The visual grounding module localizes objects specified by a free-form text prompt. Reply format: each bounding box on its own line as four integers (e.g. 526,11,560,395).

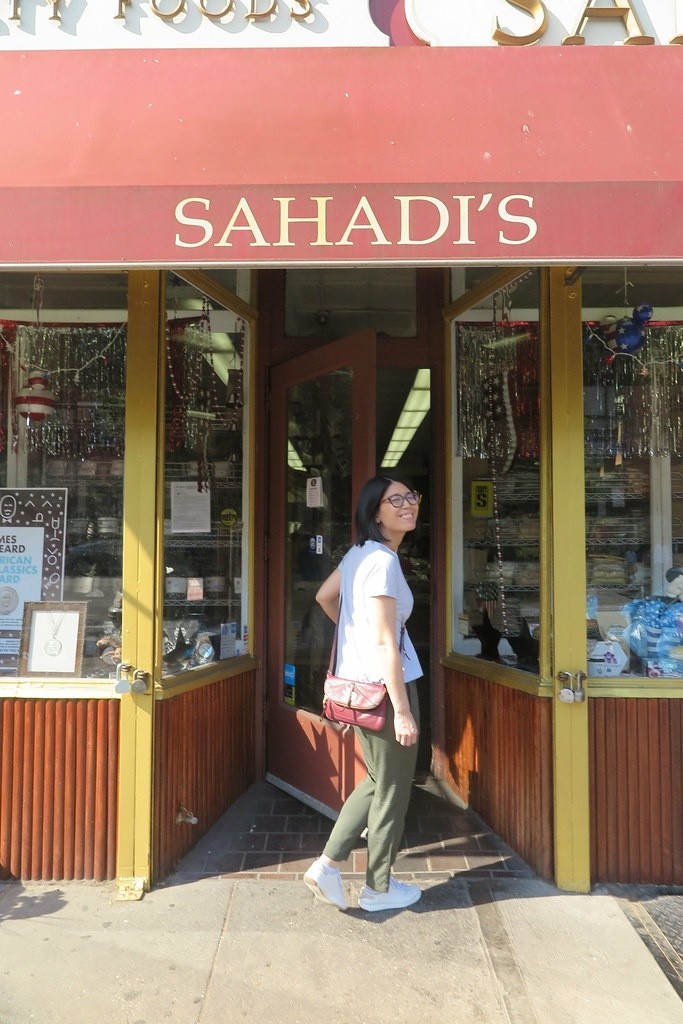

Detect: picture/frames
17,601,89,677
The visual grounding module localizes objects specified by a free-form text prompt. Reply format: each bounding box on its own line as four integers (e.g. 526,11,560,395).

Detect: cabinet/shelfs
36,458,683,636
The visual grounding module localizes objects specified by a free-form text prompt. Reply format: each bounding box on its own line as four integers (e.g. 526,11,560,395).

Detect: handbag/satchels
319,675,389,732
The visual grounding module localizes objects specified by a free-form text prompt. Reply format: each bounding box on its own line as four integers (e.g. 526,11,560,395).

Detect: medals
43,637,62,657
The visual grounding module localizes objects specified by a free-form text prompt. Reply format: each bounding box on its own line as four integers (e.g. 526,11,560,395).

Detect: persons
303,473,424,912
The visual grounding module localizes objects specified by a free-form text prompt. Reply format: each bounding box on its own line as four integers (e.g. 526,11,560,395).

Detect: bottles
43,456,240,602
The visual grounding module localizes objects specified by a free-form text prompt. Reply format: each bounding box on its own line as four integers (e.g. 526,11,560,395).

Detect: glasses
379,491,421,508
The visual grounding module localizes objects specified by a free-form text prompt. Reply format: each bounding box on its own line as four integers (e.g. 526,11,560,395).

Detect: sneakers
302,861,347,911
358,876,422,912
